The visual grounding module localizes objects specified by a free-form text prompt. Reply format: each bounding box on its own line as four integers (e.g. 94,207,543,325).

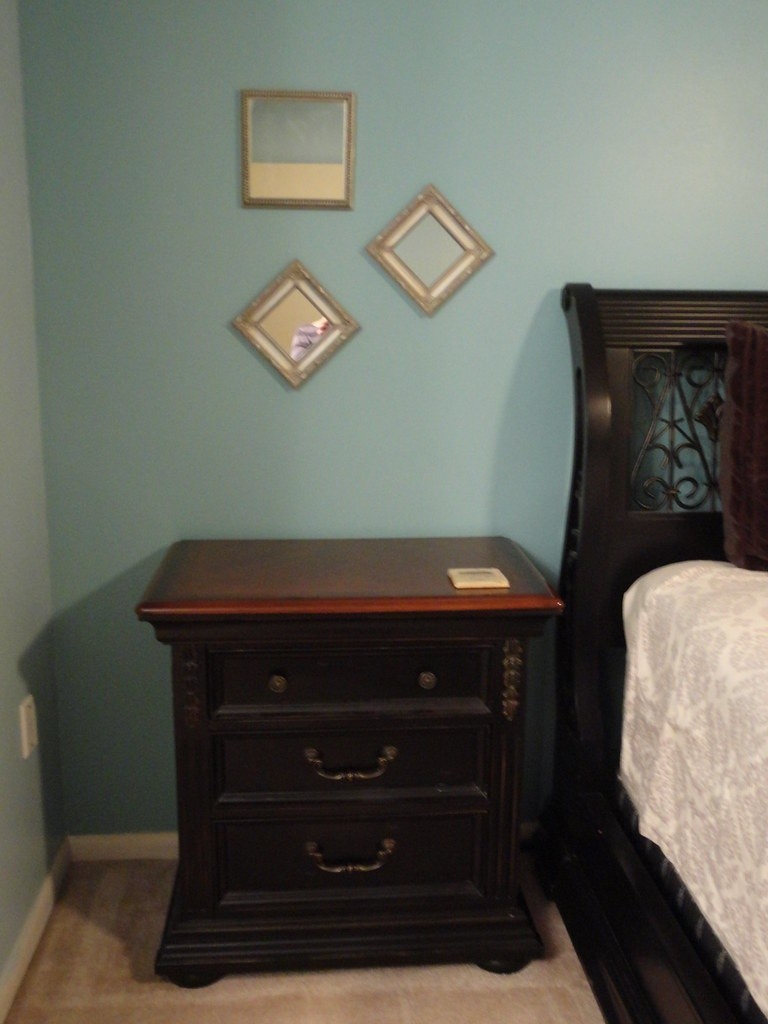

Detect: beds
555,280,767,1024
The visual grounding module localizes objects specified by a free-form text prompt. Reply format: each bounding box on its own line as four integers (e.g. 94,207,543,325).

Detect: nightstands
134,535,567,988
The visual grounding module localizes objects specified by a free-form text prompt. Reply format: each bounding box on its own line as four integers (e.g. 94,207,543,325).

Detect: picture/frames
238,87,355,211
229,256,362,391
364,182,496,318
